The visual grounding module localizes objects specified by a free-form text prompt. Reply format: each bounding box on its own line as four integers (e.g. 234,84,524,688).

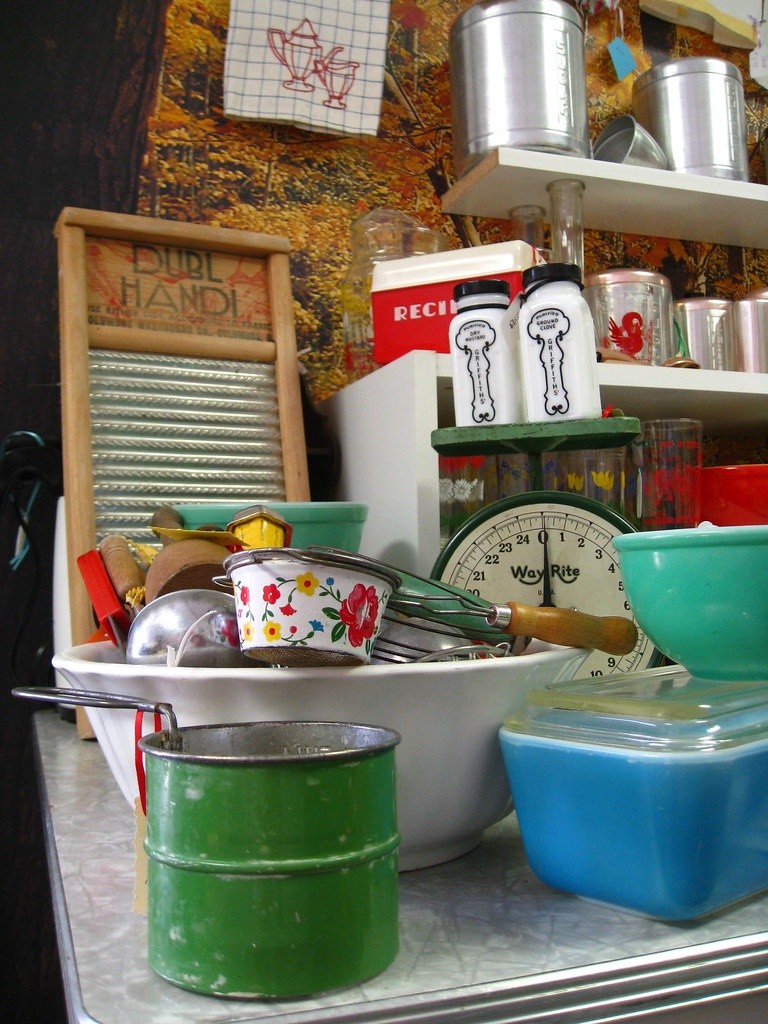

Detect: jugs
342,203,449,385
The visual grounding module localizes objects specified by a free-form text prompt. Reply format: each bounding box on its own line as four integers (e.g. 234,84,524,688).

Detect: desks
33,707,767,1024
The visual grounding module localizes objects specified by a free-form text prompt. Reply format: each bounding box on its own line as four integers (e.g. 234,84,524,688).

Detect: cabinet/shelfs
316,147,767,579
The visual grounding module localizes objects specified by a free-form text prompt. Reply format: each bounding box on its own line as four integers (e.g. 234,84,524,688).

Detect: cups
436,417,705,538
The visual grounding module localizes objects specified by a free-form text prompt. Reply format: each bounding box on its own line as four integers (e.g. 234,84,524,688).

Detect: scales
430,417,667,680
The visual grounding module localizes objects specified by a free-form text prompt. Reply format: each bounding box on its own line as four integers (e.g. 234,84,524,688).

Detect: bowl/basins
370,616,473,665
703,464,768,526
50,642,598,874
170,500,369,556
124,590,235,667
611,522,768,684
222,547,395,667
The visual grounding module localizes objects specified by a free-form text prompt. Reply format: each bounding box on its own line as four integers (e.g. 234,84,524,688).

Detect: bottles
518,262,603,424
443,280,524,426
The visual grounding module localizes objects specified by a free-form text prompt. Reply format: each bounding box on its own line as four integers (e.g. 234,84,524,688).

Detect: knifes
76,550,128,664
